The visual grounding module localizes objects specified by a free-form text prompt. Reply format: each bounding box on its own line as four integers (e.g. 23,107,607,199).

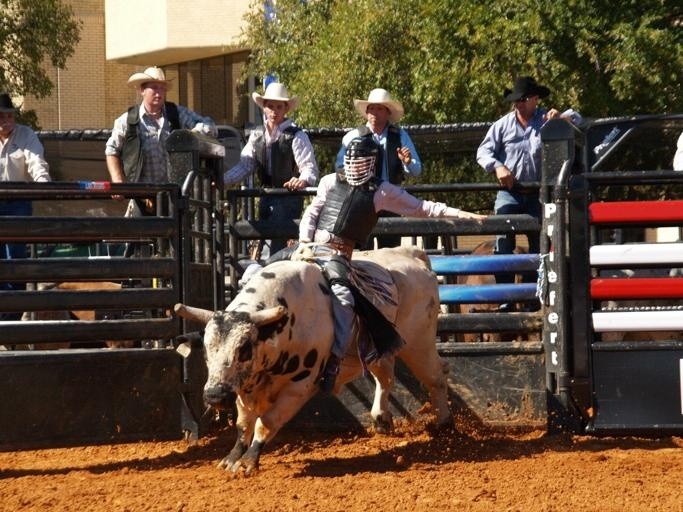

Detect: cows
15,282,134,350
174,246,454,475
457,240,542,342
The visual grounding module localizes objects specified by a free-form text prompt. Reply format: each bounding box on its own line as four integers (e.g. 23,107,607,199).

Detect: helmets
344,137,378,185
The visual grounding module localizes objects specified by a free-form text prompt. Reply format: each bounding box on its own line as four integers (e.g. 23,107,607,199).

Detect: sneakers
322,353,340,391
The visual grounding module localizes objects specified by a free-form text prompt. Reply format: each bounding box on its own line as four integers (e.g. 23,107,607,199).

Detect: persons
335,88,422,250
475,76,583,311
268,135,488,397
104,66,220,318
209,82,320,263
0,94,51,322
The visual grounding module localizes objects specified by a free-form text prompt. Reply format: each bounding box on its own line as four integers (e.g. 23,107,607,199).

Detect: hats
1,93,23,114
127,67,175,88
355,89,404,120
252,83,298,107
504,76,550,101
